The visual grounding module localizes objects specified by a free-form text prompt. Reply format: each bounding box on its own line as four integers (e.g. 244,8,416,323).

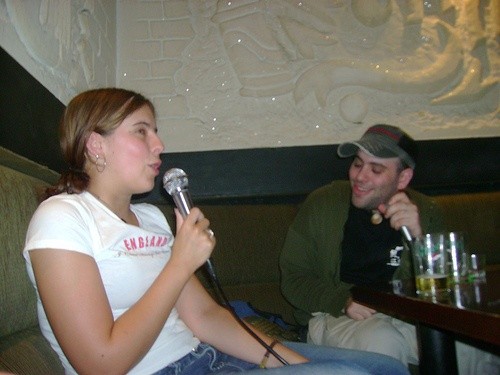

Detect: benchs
0,144,500,375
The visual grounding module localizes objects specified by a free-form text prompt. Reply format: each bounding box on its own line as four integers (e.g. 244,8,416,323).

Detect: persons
279,123,500,375
22,87,410,375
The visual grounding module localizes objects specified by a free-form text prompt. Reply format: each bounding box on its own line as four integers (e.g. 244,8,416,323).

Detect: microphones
386,201,414,247
162,168,217,279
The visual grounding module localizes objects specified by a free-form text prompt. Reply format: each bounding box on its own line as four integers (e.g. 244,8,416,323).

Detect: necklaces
90,192,128,224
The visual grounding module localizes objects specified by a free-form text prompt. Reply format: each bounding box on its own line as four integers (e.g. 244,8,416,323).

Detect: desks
351,261,500,375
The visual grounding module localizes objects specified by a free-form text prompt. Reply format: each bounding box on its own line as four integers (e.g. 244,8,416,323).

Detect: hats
338,124,420,174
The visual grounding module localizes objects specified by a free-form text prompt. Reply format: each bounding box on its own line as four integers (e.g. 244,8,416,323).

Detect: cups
411,233,487,310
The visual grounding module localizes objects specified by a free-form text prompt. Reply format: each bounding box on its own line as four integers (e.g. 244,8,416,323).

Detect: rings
207,228,214,237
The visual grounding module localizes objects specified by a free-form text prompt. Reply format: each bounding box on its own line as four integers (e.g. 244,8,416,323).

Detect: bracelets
260,340,278,368
